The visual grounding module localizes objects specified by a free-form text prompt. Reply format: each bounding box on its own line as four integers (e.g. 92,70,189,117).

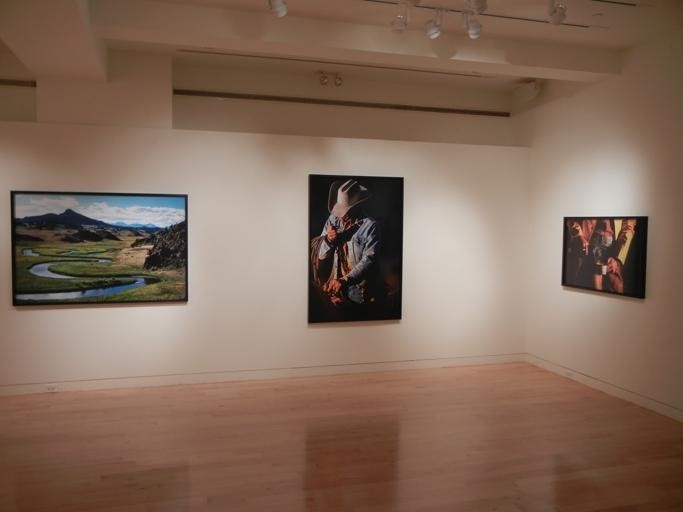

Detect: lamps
314,68,343,88
265,0,567,44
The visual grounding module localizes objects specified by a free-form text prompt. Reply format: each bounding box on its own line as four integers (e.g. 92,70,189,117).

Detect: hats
596,220,614,234
327,179,374,218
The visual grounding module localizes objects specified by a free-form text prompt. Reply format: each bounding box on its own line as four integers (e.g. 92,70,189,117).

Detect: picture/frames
305,173,405,324
559,215,648,301
7,188,191,307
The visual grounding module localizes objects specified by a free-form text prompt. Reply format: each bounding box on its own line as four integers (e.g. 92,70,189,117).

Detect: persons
565,218,636,293
309,179,398,319
